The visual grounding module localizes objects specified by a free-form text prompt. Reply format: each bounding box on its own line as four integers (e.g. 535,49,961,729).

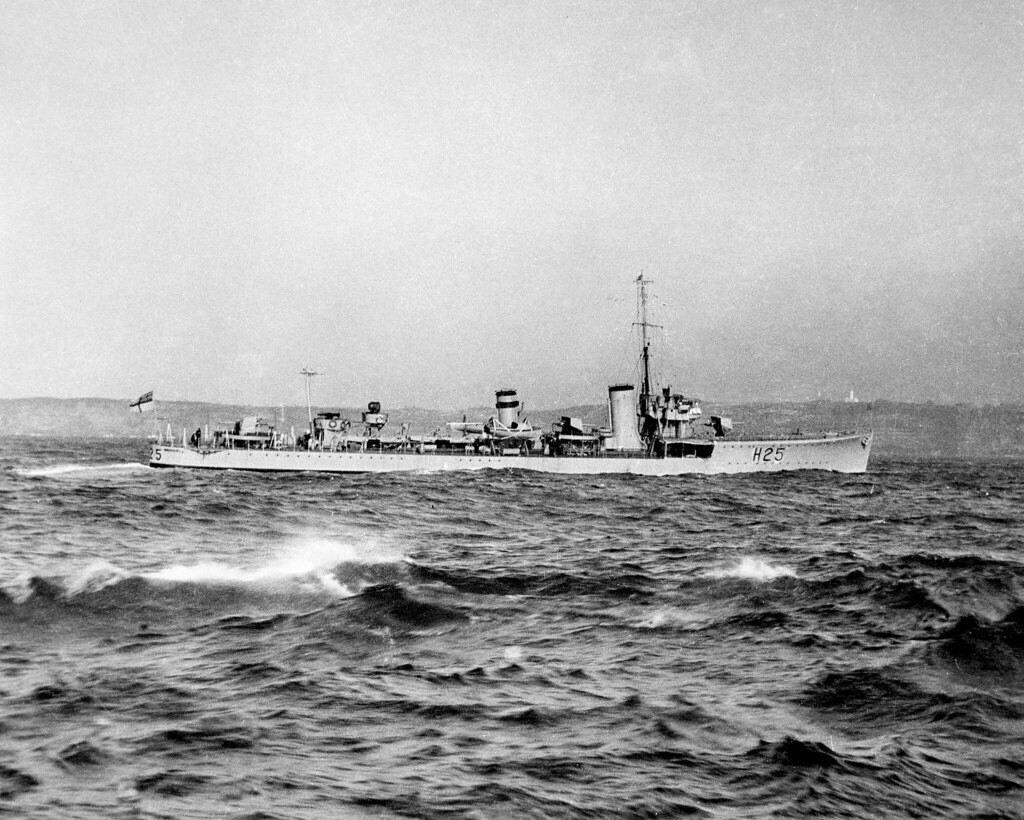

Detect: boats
128,266,873,476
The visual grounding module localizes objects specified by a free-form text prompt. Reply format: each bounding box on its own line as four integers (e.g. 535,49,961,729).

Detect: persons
191,426,201,448
297,432,310,449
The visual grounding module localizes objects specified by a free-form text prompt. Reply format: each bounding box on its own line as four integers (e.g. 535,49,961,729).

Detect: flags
130,391,154,414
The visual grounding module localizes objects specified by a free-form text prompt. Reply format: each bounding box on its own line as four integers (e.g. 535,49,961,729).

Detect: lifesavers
329,421,338,428
340,422,345,430
345,422,351,430
